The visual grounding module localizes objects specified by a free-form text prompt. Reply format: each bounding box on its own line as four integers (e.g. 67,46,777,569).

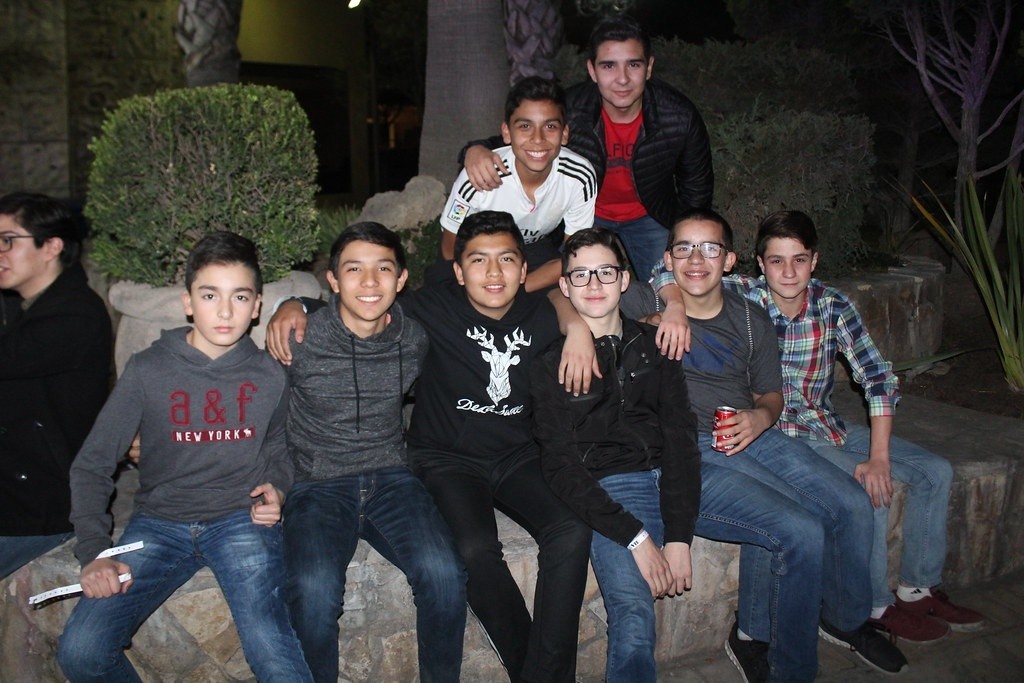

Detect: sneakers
868,590,986,643
724,611,770,683
817,610,909,675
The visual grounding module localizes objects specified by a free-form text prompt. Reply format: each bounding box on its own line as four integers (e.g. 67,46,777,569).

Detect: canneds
711,406,737,452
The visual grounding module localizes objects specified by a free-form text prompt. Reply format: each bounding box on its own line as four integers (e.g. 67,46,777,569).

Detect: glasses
560,265,625,287
665,242,730,260
1,234,40,253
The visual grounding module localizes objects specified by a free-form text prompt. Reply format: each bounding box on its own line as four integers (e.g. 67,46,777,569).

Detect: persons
267,211,665,683
440,18,715,281
266,220,469,683
0,190,117,583
650,210,987,646
56,231,314,683
528,226,824,683
546,207,910,683
